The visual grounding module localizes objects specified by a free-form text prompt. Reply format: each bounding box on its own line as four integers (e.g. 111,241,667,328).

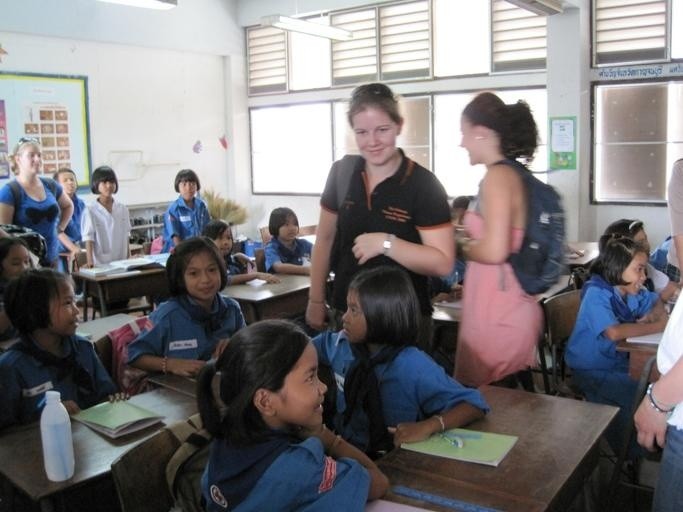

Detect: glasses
17,137,39,145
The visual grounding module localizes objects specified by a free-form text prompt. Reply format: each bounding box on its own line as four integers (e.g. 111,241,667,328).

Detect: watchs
383,233,393,255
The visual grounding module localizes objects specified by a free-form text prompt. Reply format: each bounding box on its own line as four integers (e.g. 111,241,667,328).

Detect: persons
448,90,548,395
301,79,459,359
424,195,473,307
0,134,315,432
194,267,492,511
563,160,682,512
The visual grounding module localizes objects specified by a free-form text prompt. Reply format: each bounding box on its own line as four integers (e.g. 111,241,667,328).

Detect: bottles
38,389,74,482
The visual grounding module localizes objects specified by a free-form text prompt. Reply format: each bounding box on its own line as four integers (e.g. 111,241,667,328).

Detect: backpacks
163,414,216,511
492,159,565,296
106,315,148,394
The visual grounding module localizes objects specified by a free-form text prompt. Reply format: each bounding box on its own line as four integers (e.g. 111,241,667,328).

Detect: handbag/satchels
0,224,47,260
